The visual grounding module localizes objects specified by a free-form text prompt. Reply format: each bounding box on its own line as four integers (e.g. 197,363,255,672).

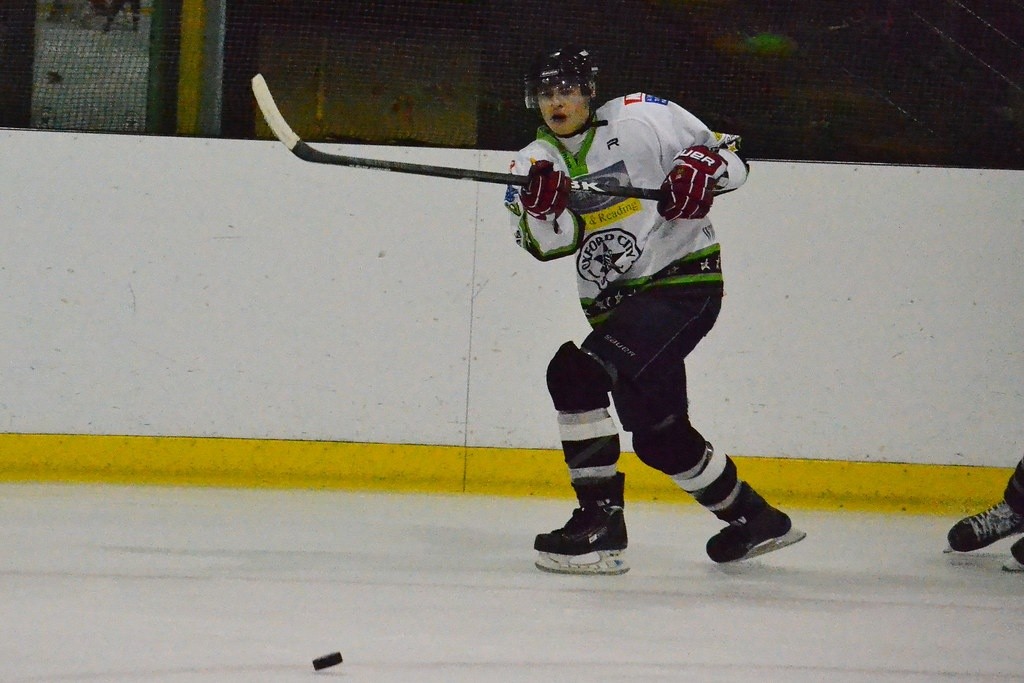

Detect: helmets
524,44,600,108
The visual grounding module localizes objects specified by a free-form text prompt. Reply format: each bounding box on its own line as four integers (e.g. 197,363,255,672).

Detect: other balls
309,649,346,673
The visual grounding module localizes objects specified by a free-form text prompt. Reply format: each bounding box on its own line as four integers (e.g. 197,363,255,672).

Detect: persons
941,455,1024,573
505,45,808,577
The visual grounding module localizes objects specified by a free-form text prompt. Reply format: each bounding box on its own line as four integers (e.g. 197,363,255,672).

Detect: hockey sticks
250,71,662,202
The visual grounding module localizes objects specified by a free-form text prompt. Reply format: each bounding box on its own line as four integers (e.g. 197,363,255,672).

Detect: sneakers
707,484,806,563
946,503,1024,577
534,472,628,575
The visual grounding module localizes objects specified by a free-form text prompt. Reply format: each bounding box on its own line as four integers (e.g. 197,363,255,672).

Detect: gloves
519,160,572,223
657,146,726,222
